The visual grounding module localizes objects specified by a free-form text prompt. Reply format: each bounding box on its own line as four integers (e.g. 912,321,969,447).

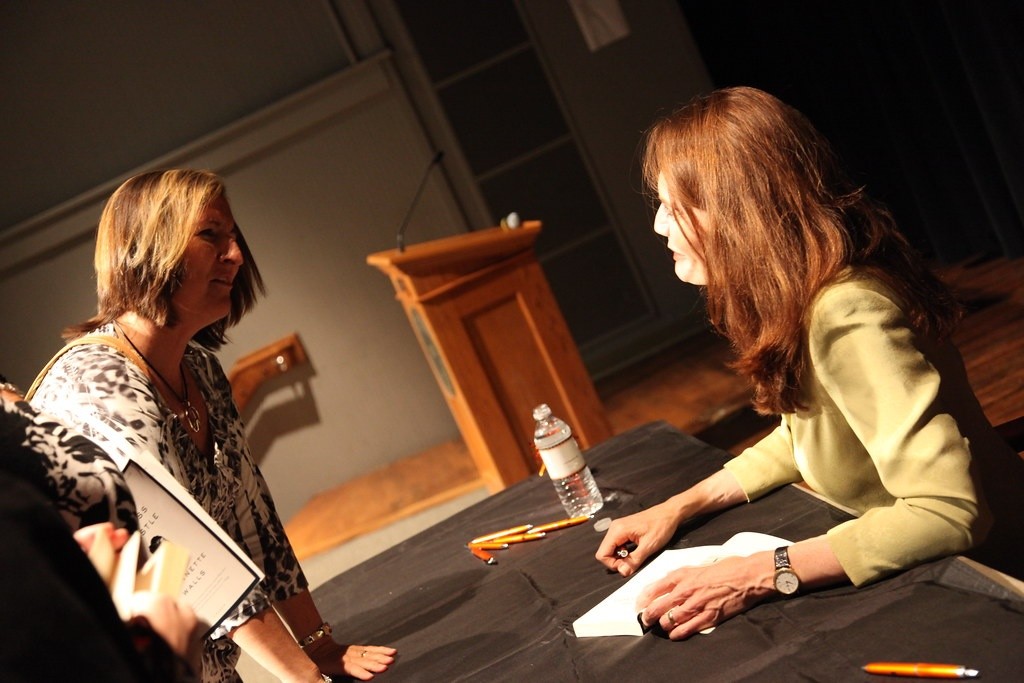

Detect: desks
307,421,1024,683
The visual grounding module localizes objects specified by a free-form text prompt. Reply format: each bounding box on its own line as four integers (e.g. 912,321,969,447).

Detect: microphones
398,150,444,252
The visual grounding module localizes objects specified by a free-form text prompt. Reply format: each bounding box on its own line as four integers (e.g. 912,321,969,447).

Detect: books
571,532,796,639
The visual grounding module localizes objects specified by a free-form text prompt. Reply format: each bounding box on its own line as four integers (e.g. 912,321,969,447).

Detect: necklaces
113,319,201,433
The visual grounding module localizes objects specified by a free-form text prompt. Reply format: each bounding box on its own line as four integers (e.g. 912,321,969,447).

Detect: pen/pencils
491,532,546,542
463,542,509,549
528,514,595,533
469,523,533,544
472,547,495,565
617,545,628,558
862,662,980,678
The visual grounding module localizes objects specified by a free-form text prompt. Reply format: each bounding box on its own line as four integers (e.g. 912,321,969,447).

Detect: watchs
773,546,804,598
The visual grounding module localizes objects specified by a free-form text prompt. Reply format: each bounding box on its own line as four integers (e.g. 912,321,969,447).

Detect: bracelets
321,673,332,683
298,622,332,647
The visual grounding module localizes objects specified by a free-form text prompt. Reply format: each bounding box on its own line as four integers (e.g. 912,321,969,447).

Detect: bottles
533,403,604,518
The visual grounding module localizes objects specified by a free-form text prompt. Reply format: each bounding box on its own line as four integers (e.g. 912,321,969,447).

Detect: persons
595,87,1024,641
27,168,397,683
0,378,211,683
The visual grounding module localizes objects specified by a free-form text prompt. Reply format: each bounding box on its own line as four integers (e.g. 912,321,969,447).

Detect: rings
361,650,367,658
668,610,675,624
